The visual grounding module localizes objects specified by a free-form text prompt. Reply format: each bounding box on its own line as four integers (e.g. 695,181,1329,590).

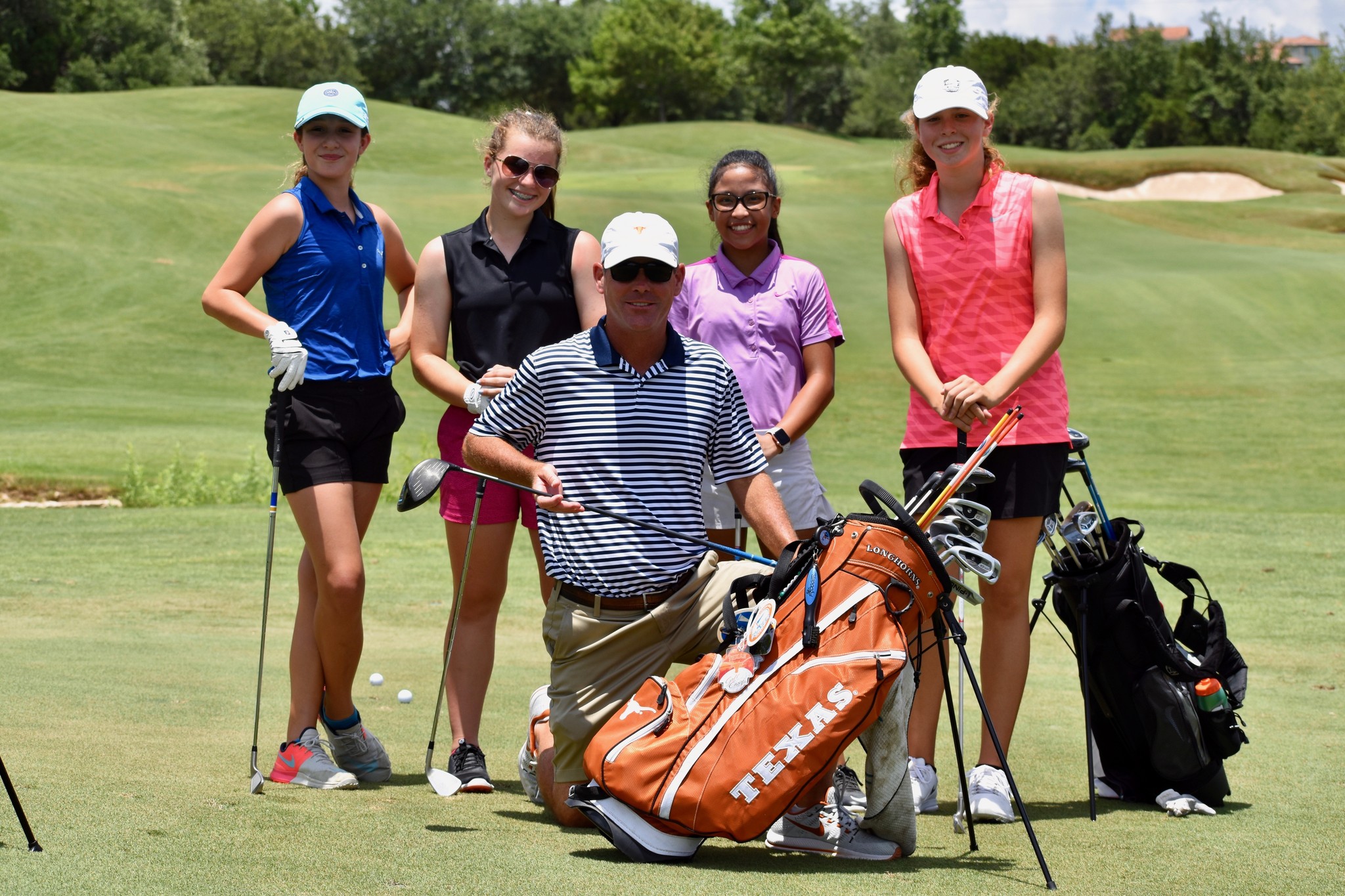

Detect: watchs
767,427,792,453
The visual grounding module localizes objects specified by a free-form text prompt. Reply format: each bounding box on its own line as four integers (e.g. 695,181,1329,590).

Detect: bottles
1194,678,1231,712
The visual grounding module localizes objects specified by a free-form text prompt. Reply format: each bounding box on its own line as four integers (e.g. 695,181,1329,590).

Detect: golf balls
368,673,384,687
398,689,413,704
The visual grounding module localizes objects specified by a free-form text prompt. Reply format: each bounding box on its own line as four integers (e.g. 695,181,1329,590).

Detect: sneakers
764,770,901,861
832,756,867,813
964,765,1015,824
447,738,494,793
518,683,551,804
270,727,359,789
316,686,392,782
906,756,938,814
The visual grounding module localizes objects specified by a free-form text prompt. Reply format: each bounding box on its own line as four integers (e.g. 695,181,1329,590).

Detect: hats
600,212,679,268
294,82,369,135
913,65,989,121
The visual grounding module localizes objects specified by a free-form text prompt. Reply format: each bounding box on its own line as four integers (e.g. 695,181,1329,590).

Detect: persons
667,150,868,812
464,211,902,861
202,81,417,792
884,65,1070,822
409,103,609,792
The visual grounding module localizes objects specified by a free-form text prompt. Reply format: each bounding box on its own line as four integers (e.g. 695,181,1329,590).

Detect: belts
553,560,702,611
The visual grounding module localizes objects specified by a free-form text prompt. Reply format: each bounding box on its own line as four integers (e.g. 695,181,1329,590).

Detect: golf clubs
731,493,745,565
1035,426,1118,575
393,455,783,569
423,472,488,800
949,422,973,837
246,365,289,797
893,461,1003,607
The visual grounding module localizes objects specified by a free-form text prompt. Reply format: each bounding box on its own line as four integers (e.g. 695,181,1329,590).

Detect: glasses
609,260,672,282
710,192,777,212
491,156,560,188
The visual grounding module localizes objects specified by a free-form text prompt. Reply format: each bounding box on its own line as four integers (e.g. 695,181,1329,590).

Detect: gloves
464,383,501,416
264,322,308,392
